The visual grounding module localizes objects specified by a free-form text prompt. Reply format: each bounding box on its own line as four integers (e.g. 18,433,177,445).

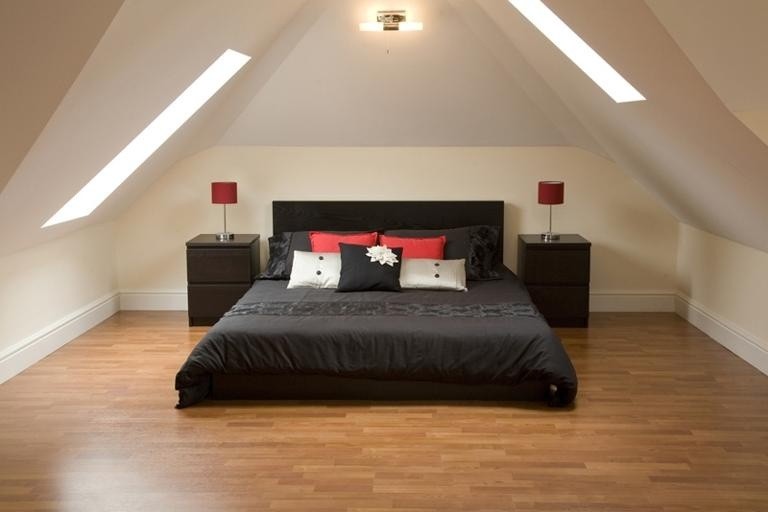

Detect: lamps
211,182,238,241
538,180,564,240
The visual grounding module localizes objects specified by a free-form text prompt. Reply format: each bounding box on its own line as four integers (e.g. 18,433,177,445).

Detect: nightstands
515,232,591,328
185,233,260,327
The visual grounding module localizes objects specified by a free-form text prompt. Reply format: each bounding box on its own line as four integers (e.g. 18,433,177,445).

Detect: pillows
256,224,501,293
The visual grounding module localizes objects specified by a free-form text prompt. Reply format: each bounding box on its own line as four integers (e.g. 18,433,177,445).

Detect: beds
208,201,567,416
